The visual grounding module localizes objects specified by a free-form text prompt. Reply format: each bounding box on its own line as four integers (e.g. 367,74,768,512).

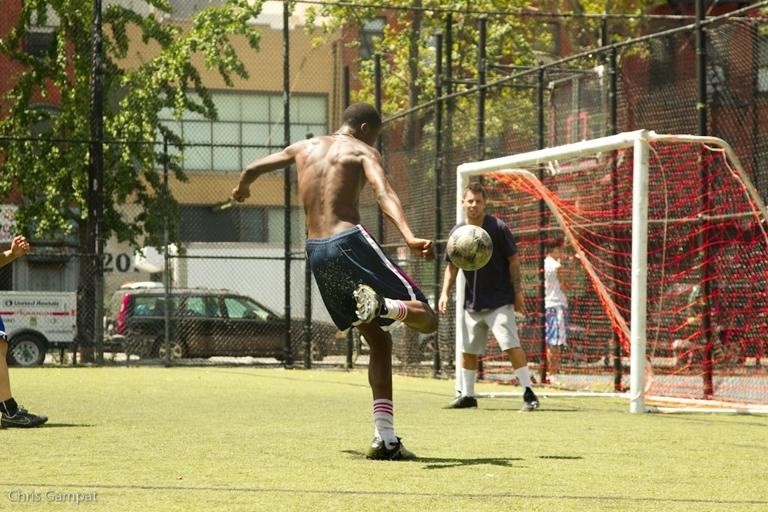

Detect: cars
388,298,434,362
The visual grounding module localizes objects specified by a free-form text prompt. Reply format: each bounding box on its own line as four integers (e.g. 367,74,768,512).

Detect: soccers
448,224,493,270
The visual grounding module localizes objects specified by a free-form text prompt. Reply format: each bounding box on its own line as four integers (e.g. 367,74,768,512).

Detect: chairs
206,300,219,317
156,298,175,316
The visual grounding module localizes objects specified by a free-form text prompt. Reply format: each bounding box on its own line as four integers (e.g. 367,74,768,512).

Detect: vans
102,287,336,358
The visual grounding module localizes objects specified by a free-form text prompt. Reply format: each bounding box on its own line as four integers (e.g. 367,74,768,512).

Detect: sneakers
521,387,539,411
365,436,416,460
1,409,48,427
353,283,388,324
442,395,477,409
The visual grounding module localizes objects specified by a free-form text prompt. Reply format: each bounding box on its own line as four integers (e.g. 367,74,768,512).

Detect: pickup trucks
0,289,77,368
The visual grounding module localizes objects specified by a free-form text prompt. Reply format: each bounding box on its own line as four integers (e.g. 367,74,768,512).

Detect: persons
671,284,704,368
438,181,540,412
544,237,574,391
0,234,48,427
231,102,437,460
